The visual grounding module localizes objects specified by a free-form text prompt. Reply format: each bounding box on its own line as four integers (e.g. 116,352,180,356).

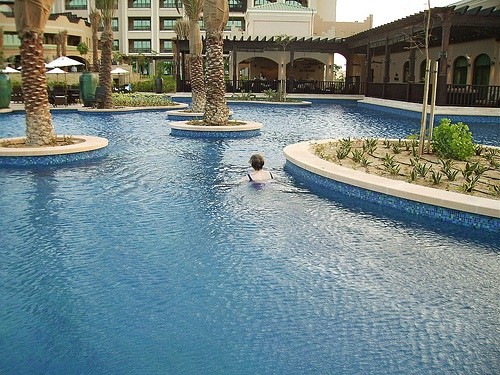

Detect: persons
248,71,355,94
393,72,400,83
237,154,284,192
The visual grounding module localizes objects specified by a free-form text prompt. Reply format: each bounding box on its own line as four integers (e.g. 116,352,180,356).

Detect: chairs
86,87,107,109
12,85,24,104
48,85,81,108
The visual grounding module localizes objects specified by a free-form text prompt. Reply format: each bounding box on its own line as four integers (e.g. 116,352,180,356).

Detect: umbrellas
46,68,69,85
46,54,85,87
110,67,130,86
0,66,21,92
